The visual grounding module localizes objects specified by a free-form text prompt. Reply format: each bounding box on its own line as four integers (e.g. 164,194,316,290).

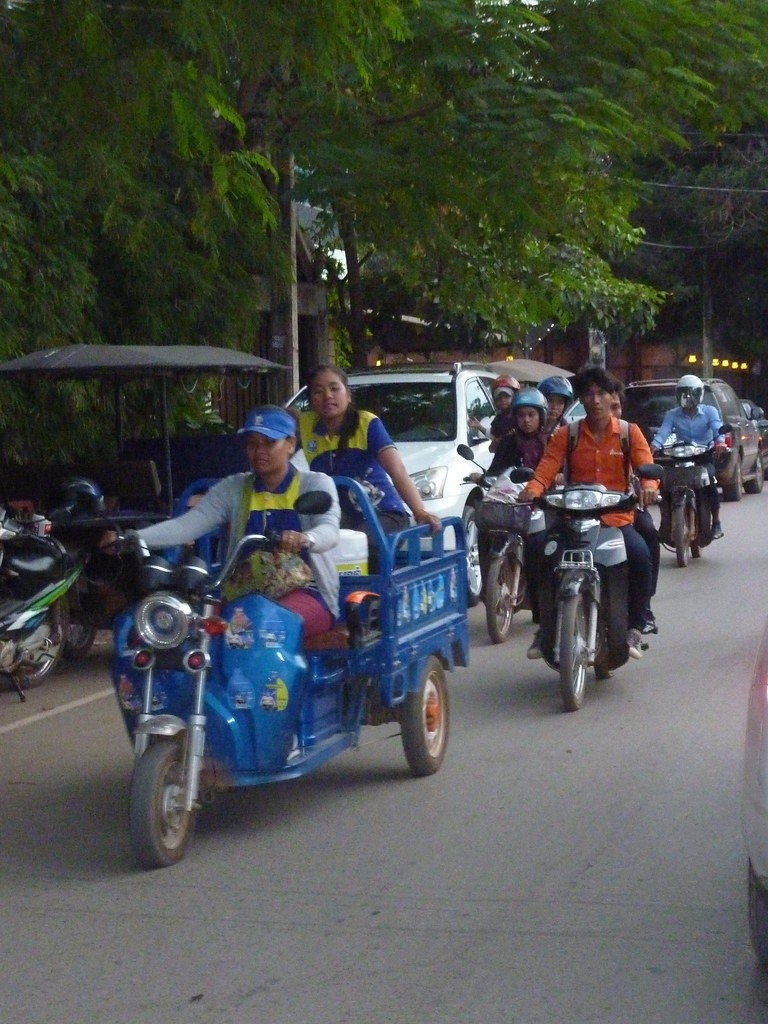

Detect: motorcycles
635,423,734,568
453,440,665,712
62,477,468,871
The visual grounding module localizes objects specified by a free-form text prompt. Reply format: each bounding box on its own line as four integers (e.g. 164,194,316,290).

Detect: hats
494,387,513,398
237,407,296,441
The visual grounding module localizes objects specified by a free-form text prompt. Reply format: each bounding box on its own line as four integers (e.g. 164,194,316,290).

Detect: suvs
620,377,763,502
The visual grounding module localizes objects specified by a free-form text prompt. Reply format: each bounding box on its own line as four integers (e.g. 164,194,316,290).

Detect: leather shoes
711,521,723,538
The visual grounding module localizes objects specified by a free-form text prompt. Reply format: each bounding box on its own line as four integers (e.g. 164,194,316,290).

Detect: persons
648,374,728,538
96,405,342,762
514,363,661,663
281,365,442,577
467,369,572,602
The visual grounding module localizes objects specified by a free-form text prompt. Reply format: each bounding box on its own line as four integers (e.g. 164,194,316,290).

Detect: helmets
538,376,574,405
491,375,521,399
675,375,705,408
60,475,100,499
512,386,549,427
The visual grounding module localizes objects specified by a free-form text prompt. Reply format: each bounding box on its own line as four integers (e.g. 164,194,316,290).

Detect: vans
276,359,512,610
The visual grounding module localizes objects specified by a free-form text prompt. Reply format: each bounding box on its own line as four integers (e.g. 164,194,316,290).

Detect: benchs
305,590,379,651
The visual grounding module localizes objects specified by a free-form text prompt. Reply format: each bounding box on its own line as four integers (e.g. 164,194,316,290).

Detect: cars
739,399,768,480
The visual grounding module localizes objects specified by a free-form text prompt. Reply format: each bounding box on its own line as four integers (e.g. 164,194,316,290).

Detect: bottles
436,574,444,609
117,674,142,716
226,608,254,650
151,678,169,714
420,581,428,614
396,596,403,626
428,580,436,612
402,585,411,624
227,668,255,709
259,671,289,711
449,568,458,603
118,615,141,657
412,583,421,619
258,608,287,649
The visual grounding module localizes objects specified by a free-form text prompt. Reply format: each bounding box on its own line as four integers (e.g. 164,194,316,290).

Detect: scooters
0,511,93,707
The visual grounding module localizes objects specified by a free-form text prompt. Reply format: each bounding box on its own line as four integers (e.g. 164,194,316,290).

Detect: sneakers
527,633,544,659
627,628,643,660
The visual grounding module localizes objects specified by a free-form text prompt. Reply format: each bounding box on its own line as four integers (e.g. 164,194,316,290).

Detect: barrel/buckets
329,527,369,576
